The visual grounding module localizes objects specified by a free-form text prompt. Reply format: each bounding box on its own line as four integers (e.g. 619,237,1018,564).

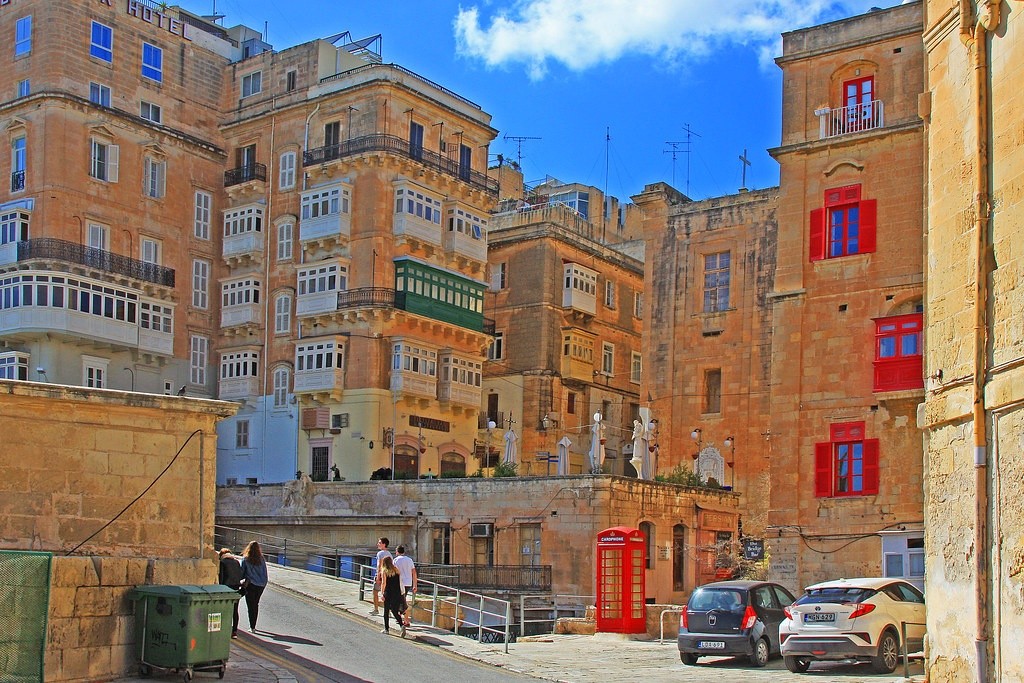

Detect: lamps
37,368,50,382
542,415,550,428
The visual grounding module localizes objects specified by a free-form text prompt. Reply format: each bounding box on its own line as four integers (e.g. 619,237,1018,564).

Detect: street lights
486,421,496,478
690,428,702,486
648,418,660,480
124,367,134,391
723,435,735,492
593,409,603,474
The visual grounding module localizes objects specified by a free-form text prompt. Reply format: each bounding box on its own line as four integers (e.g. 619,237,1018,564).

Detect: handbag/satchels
238,580,246,597
400,576,406,596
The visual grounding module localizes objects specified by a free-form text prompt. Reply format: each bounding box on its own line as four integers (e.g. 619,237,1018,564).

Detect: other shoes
380,628,389,635
400,627,405,639
248,627,256,634
402,620,410,627
369,610,379,615
231,631,238,639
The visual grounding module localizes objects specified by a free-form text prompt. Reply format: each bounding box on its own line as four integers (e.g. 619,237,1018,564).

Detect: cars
677,579,800,667
779,577,926,674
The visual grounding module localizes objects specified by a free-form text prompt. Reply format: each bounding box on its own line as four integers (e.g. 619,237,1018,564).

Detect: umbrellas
505,429,518,463
558,436,571,475
589,422,605,473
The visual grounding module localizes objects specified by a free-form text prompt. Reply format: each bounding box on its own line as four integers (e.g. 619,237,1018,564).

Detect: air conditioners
471,524,493,537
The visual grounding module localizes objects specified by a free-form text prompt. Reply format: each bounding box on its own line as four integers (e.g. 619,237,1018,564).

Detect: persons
392,545,417,627
369,537,393,617
240,541,268,633
219,548,241,639
632,420,644,458
381,556,406,638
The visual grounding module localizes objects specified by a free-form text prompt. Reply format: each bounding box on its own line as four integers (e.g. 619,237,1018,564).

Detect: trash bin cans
419,474,437,479
128,584,241,683
